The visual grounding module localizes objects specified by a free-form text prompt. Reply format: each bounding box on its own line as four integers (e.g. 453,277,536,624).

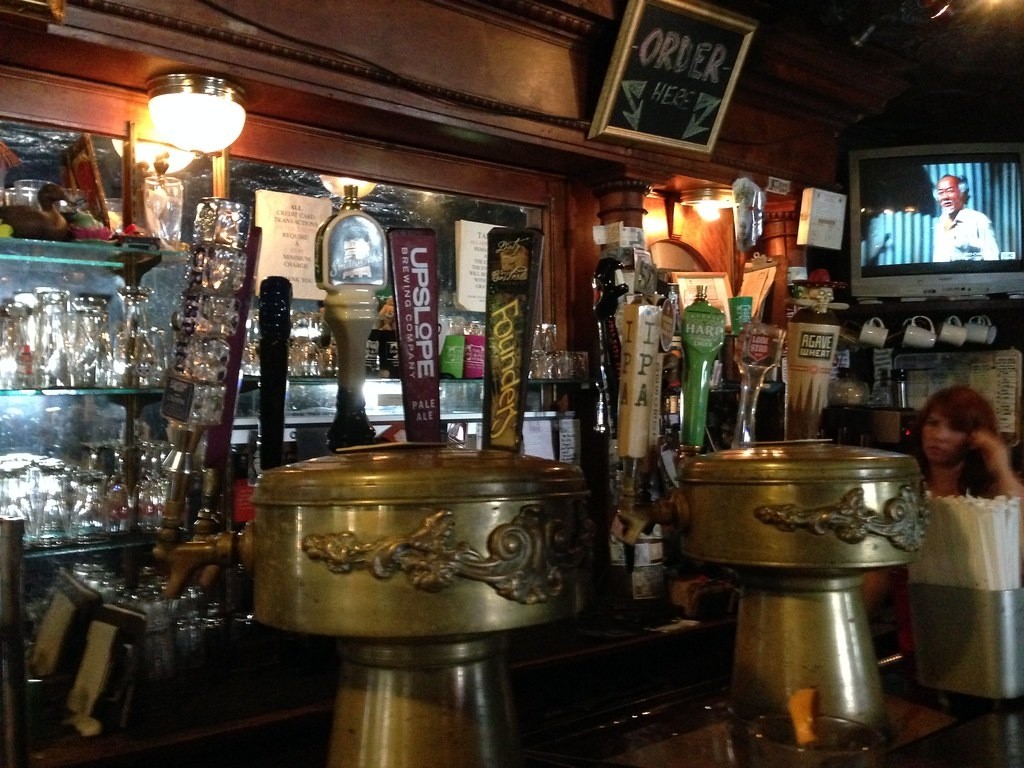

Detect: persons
863,385,1024,696
932,174,999,262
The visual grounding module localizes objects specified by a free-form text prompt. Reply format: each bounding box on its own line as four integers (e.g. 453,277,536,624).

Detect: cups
0,286,222,690
964,316,990,344
143,176,184,252
890,367,909,409
859,317,889,349
902,316,937,349
982,316,997,344
0,178,86,211
938,316,968,348
746,714,885,767
528,323,589,380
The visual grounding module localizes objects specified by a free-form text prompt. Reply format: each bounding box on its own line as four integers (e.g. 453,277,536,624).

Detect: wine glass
239,306,338,377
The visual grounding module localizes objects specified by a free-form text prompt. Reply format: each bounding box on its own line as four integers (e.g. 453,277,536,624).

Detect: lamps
145,72,247,153
680,188,734,222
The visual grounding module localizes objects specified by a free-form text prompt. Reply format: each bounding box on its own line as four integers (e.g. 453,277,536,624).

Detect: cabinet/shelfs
1,124,919,767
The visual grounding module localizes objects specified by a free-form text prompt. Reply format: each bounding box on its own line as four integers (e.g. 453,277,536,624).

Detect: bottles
438,314,485,380
828,365,870,407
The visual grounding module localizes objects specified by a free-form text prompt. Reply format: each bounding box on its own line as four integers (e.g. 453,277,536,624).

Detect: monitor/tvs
846,141,1024,298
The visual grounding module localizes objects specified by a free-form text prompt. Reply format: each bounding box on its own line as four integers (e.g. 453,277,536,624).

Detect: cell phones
944,444,976,471
